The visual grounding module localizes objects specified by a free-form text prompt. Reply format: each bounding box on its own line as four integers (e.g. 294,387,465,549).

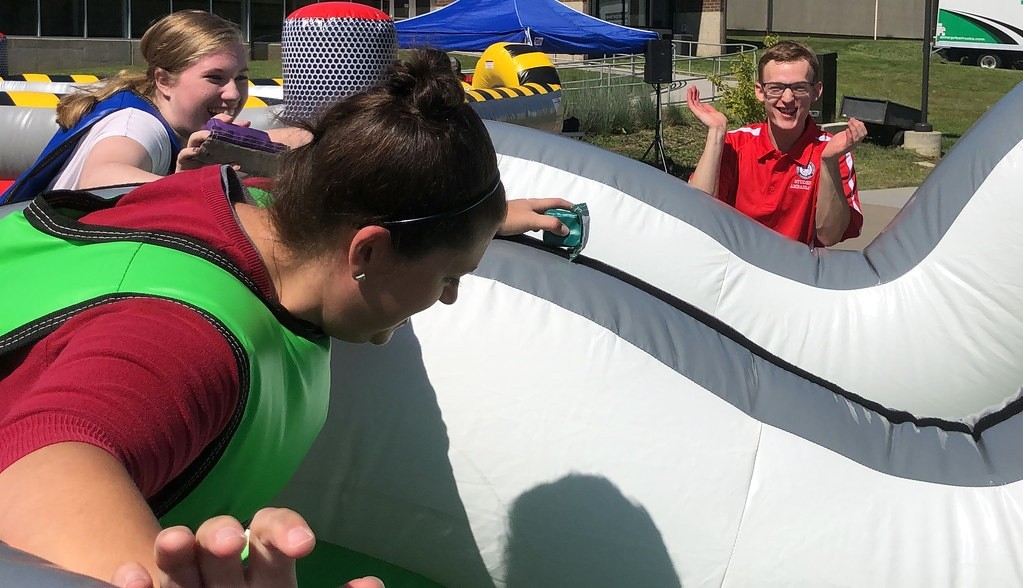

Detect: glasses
761,80,816,98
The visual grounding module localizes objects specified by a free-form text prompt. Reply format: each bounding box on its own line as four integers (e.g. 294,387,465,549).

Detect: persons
0,7,310,207
0,43,576,588
687,39,868,247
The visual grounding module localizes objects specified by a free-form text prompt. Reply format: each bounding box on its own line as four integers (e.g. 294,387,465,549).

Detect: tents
395,0,659,64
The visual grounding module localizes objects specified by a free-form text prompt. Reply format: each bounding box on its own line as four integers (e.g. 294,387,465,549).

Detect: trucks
932,0,1023,68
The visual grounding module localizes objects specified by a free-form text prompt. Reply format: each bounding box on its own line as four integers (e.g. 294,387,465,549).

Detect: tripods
639,84,668,174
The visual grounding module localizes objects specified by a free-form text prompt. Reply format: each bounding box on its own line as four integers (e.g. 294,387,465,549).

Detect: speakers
644,39,673,84
